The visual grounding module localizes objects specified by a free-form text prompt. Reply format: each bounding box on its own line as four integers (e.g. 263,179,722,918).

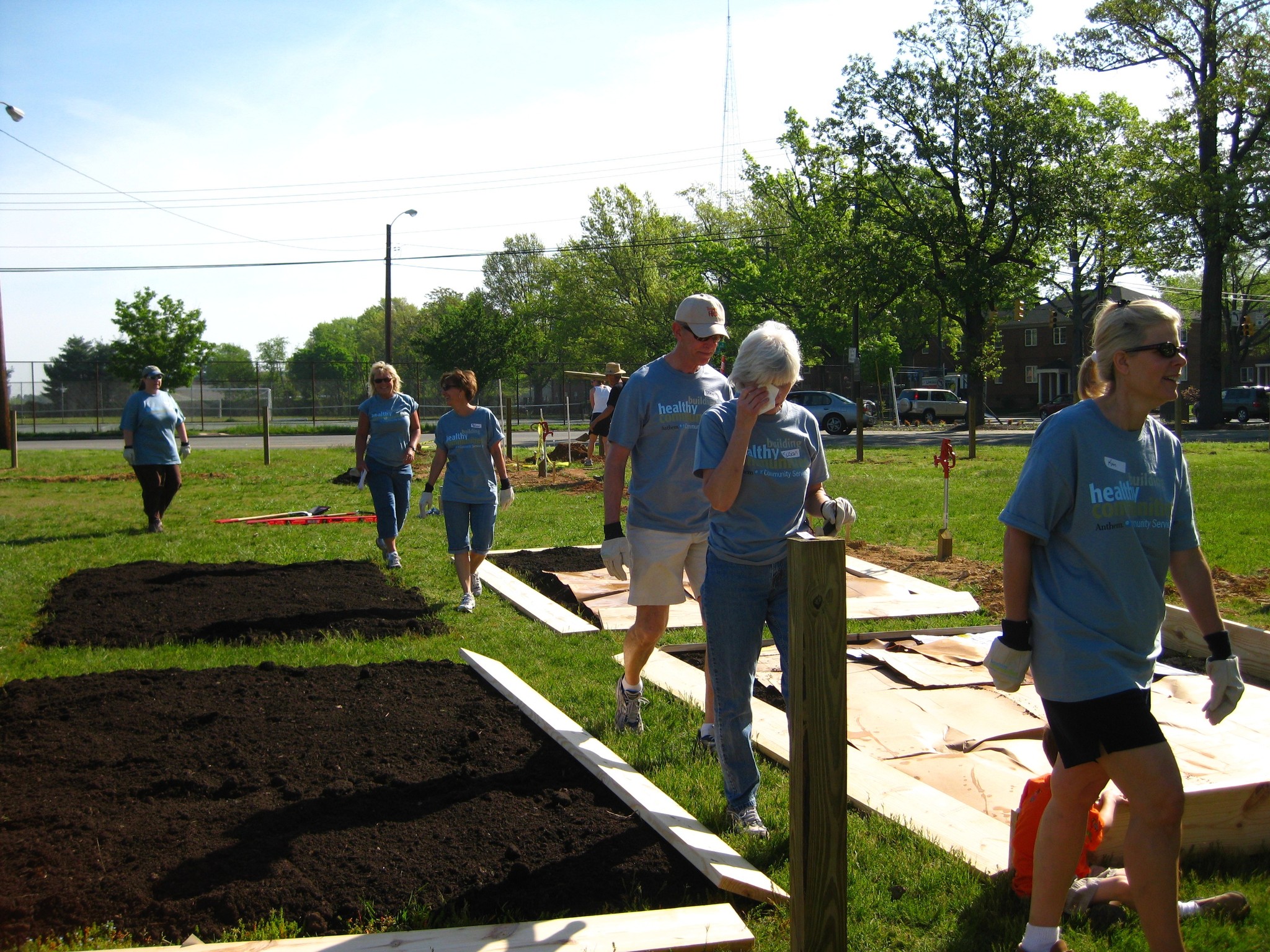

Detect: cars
1038,393,1073,421
785,390,875,436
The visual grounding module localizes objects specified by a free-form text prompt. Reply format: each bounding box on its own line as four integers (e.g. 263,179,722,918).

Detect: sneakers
615,672,769,839
376,537,482,614
584,459,593,466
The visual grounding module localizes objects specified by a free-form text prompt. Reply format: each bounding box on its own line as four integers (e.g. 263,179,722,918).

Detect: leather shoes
1188,891,1247,916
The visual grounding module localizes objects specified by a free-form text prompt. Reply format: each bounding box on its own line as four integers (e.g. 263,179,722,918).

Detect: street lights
1066,261,1105,319
384,209,419,366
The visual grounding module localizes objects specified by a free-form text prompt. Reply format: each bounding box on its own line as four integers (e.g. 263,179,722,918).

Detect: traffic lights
1245,322,1254,336
1240,316,1249,337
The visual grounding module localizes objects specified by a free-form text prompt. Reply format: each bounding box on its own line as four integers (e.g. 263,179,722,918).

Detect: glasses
374,378,394,383
145,375,162,380
1122,341,1189,360
605,374,609,376
683,325,724,342
804,494,838,539
591,380,595,383
437,384,456,392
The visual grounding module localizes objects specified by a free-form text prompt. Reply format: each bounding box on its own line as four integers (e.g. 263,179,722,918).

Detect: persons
983,298,1245,952
583,371,612,466
119,365,191,534
692,319,856,839
593,362,627,482
418,368,515,614
599,294,737,751
355,360,421,570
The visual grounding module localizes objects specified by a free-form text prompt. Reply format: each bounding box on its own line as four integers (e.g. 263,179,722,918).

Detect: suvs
893,389,968,425
1191,385,1270,424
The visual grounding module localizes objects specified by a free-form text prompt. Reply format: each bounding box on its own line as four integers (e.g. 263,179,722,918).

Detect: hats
142,365,164,377
602,362,626,374
675,293,730,339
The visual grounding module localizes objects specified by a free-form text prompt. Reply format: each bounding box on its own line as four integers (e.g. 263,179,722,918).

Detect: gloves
179,441,191,460
123,446,135,464
821,497,856,530
500,478,514,511
983,619,1033,693
419,483,434,519
601,521,631,581
1202,630,1245,725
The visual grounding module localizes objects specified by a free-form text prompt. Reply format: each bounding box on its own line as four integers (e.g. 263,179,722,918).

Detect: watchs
409,446,417,453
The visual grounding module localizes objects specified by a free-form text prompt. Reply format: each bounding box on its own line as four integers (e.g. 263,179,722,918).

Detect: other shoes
1016,940,1069,952
593,475,604,481
148,519,164,533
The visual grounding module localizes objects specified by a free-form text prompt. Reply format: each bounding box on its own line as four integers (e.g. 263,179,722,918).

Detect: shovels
215,506,331,523
276,510,375,519
575,433,590,442
